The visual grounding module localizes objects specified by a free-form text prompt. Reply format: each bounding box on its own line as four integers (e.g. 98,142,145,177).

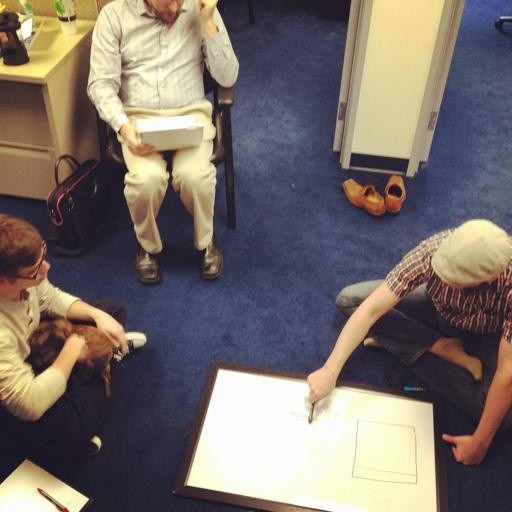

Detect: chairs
96,5,235,229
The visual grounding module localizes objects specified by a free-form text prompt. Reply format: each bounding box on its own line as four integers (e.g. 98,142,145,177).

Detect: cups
54,0,78,36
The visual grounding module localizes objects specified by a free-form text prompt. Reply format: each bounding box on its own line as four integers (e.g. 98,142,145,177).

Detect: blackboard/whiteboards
169,361,447,512
0,454,95,512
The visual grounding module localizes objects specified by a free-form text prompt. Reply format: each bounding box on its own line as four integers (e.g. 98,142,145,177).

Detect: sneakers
108,331,148,367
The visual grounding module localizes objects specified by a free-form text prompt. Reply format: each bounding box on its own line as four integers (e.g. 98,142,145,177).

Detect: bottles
0,12,31,67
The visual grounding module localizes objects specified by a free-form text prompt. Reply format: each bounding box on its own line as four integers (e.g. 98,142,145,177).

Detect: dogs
21,318,122,403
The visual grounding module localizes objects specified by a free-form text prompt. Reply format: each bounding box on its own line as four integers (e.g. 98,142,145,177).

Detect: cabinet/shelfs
0,12,102,201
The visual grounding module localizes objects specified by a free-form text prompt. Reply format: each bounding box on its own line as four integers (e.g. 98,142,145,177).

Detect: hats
430,218,512,291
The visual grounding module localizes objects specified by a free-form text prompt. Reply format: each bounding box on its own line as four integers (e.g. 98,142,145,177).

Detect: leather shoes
342,172,407,216
134,241,161,285
197,234,224,279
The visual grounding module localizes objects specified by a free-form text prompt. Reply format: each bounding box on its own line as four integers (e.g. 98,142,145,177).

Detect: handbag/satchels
44,153,112,251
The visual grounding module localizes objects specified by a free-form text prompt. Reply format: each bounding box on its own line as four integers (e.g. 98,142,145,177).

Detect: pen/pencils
309,404,315,423
402,386,433,392
38,488,70,512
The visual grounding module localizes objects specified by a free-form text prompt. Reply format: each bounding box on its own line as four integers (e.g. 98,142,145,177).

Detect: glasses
13,239,48,281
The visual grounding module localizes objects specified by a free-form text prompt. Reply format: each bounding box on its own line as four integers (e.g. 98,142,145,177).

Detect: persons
304,219,511,471
1,211,151,457
87,0,239,283
